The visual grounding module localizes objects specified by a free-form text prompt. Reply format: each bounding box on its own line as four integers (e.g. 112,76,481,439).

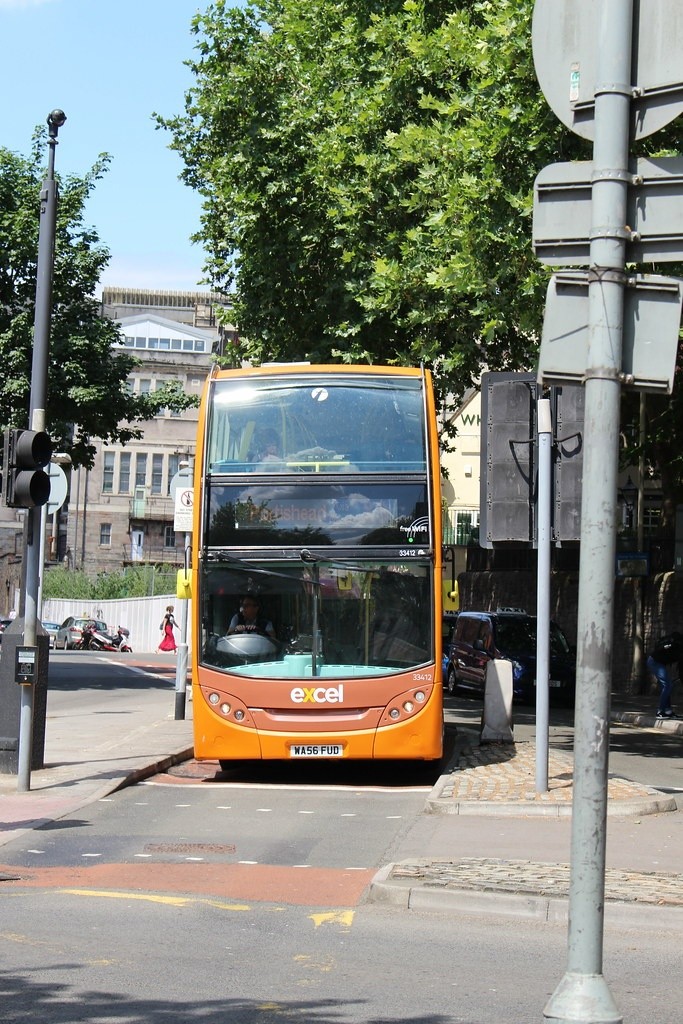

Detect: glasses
242,604,258,609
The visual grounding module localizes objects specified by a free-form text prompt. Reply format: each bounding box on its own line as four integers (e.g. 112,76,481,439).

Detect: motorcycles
72,621,98,650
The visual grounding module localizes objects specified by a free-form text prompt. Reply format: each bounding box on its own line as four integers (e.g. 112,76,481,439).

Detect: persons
155,606,182,654
82,612,87,617
225,596,276,639
113,626,132,652
358,585,420,665
252,428,283,464
646,631,683,717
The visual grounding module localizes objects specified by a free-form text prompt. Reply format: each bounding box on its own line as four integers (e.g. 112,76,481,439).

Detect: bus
177,358,460,770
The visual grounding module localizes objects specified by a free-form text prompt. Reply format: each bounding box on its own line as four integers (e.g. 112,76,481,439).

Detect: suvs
54,616,109,649
0,618,15,642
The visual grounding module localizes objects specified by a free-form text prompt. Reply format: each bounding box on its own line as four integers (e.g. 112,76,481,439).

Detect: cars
41,621,61,648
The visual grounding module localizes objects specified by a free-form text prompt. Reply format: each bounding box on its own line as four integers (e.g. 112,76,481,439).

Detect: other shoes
155,650,159,654
174,653,178,655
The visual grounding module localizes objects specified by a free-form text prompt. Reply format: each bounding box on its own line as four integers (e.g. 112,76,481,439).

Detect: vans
446,608,576,703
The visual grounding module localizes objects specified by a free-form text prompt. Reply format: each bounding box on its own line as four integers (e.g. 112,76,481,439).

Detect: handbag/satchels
160,621,163,630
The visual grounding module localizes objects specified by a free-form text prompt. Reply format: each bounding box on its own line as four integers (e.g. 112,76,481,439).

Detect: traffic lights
9,430,53,508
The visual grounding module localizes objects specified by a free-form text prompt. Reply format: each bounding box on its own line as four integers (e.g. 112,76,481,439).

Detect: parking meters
14,644,39,687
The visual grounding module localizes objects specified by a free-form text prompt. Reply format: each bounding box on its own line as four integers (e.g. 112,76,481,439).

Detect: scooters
88,626,132,652
